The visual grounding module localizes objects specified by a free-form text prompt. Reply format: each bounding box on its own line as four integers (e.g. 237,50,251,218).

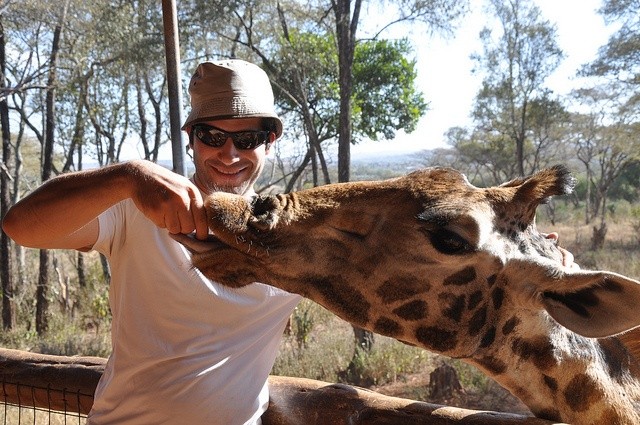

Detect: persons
1,58,586,425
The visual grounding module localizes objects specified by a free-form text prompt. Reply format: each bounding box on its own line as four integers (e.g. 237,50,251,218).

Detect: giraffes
169,165,640,425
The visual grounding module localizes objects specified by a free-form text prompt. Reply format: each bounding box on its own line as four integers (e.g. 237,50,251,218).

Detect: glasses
190,119,272,150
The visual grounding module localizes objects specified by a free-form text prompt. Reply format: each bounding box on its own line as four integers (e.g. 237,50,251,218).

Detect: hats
181,60,283,138
